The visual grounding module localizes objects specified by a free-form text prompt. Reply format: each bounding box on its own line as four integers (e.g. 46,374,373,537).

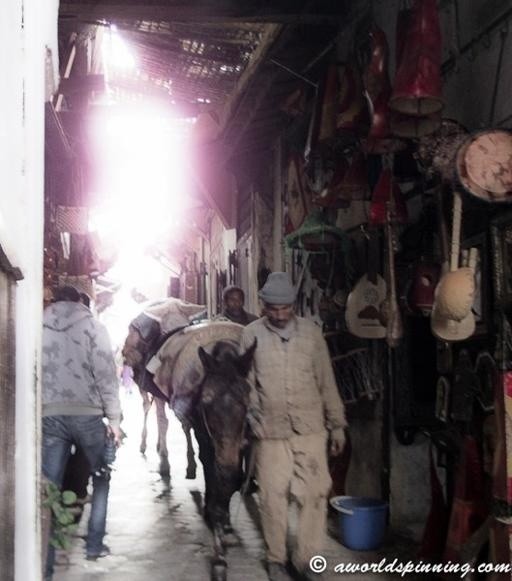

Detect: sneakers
301,566,325,581
88,546,110,560
267,562,293,581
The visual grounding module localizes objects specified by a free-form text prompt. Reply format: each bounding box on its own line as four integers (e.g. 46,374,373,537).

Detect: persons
120,361,134,395
43,287,122,581
61,293,91,524
238,273,350,581
214,287,262,500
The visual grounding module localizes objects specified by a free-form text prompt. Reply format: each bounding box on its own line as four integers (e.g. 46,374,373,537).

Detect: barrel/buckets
330,495,389,552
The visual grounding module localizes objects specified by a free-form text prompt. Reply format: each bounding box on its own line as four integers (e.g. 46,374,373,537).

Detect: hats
259,271,295,305
283,206,344,253
430,267,476,341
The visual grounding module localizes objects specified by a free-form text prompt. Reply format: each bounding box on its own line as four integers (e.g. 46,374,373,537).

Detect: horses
131,325,257,581
